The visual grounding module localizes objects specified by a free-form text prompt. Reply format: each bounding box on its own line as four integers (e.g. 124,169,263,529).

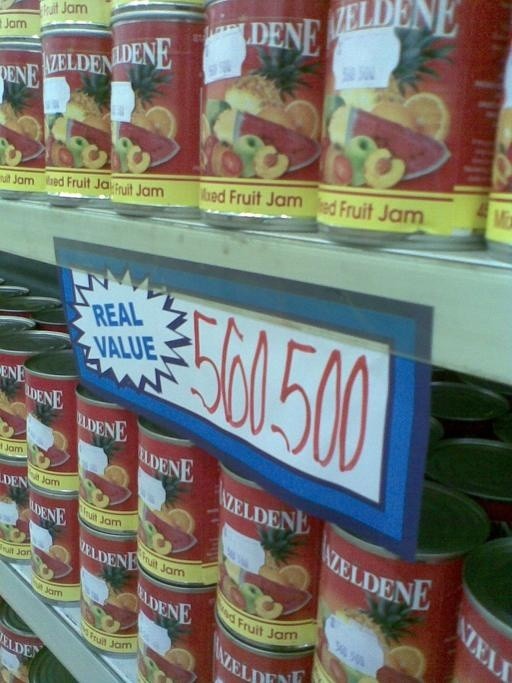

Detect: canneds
427,379,510,424
114,0,203,10
0,455,31,560
29,307,69,330
0,40,45,200
30,646,80,682
489,404,511,442
315,0,512,250
137,416,218,586
135,564,214,683
425,437,511,537
484,39,512,266
311,478,491,683
214,461,325,653
206,602,316,683
108,10,205,219
0,284,29,302
27,481,80,606
429,411,448,444
0,602,46,683
36,0,113,26
0,0,42,40
451,534,512,683
0,330,72,463
201,1,331,231
0,314,35,335
0,296,61,316
0,276,5,282
73,382,137,535
22,351,86,496
63,417,70,422
74,513,139,658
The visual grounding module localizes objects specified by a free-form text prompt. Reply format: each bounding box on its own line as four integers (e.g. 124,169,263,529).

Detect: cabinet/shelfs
0,203,511,683
